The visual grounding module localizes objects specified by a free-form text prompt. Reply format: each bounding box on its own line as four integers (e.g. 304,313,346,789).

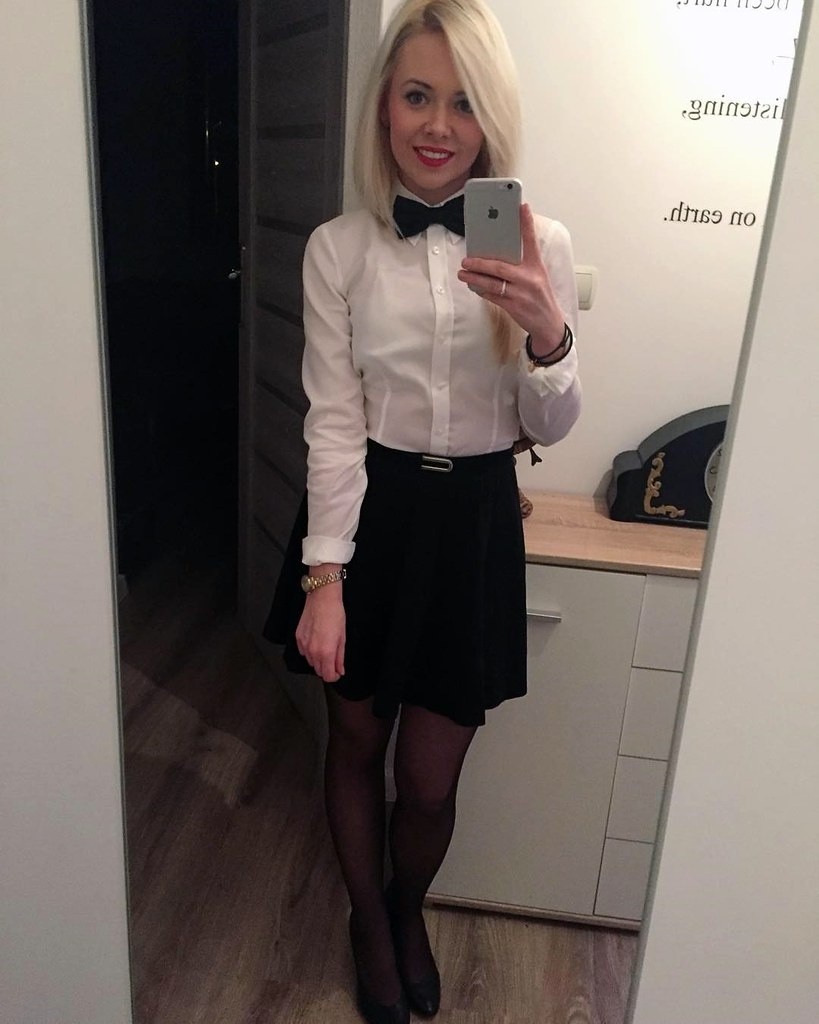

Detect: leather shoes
348,906,410,1024
383,892,441,1018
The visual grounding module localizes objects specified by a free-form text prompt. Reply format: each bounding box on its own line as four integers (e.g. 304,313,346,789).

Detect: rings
500,279,506,296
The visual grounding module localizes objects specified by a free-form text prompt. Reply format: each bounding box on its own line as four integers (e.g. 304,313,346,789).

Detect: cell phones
462,178,523,268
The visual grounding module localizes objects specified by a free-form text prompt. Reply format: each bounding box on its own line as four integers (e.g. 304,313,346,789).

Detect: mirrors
81,0,819,1024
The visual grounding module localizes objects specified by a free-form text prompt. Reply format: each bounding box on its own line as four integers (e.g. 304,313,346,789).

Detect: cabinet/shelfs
385,562,709,933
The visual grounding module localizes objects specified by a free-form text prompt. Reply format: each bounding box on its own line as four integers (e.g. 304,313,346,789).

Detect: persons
263,0,584,1024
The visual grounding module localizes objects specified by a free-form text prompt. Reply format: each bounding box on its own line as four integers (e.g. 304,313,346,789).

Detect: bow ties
392,192,465,238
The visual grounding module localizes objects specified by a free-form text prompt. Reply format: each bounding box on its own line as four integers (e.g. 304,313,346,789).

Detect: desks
511,487,706,582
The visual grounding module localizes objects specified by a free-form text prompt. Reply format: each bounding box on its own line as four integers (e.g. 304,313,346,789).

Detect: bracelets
525,323,573,369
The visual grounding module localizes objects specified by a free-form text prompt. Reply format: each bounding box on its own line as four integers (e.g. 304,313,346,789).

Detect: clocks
605,402,730,526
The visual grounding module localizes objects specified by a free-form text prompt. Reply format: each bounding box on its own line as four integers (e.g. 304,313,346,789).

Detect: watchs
301,568,346,593
